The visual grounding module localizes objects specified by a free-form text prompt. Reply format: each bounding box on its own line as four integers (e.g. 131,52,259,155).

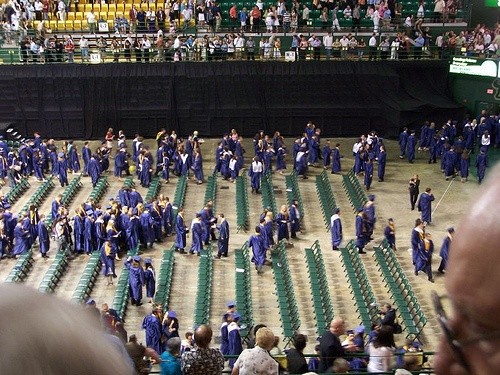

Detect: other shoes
17,59,457,63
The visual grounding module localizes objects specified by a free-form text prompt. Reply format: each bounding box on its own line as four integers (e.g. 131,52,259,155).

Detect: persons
1,0,500,375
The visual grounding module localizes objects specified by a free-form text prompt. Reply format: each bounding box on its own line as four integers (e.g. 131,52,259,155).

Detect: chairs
0,0,467,62
0,169,428,346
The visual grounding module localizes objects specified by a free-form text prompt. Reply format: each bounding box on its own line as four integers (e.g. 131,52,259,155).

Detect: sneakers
107,282,113,286
197,252,200,256
333,248,339,250
204,243,211,245
212,238,218,241
438,268,445,274
189,251,195,254
180,251,187,254
428,278,434,283
255,266,263,274
221,254,227,257
131,301,136,305
136,302,143,306
146,300,153,303
214,256,220,259
414,271,418,276
175,248,179,252
358,251,367,254
